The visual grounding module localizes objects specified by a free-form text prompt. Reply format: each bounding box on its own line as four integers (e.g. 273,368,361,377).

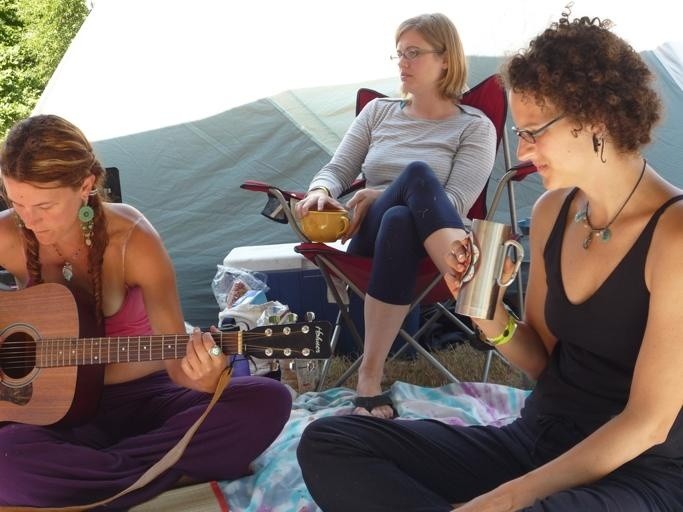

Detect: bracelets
480,316,519,347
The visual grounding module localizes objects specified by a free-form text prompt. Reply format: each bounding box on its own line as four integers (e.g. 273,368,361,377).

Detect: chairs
240,73,537,391
1,167,122,291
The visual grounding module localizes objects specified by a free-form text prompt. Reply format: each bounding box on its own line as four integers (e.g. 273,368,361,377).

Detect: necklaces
574,159,646,250
52,240,85,283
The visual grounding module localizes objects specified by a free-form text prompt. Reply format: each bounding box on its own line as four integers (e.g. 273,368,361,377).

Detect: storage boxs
225,246,420,361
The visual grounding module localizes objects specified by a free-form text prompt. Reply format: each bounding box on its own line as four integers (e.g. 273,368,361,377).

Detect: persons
296,2,682,512
296,13,498,419
0,113,292,511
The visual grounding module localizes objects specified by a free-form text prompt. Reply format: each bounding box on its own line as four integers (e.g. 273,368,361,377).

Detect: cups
299,209,350,243
452,218,526,321
279,359,320,393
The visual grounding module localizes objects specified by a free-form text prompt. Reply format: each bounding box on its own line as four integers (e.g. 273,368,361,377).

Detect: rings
207,344,224,357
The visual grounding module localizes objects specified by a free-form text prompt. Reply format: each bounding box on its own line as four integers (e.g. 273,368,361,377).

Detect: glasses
512,112,566,143
390,49,443,61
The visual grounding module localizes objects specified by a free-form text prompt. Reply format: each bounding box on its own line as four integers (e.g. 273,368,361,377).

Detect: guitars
0,284,332,426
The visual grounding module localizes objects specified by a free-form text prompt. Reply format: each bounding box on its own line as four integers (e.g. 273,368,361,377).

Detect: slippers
352,394,398,421
